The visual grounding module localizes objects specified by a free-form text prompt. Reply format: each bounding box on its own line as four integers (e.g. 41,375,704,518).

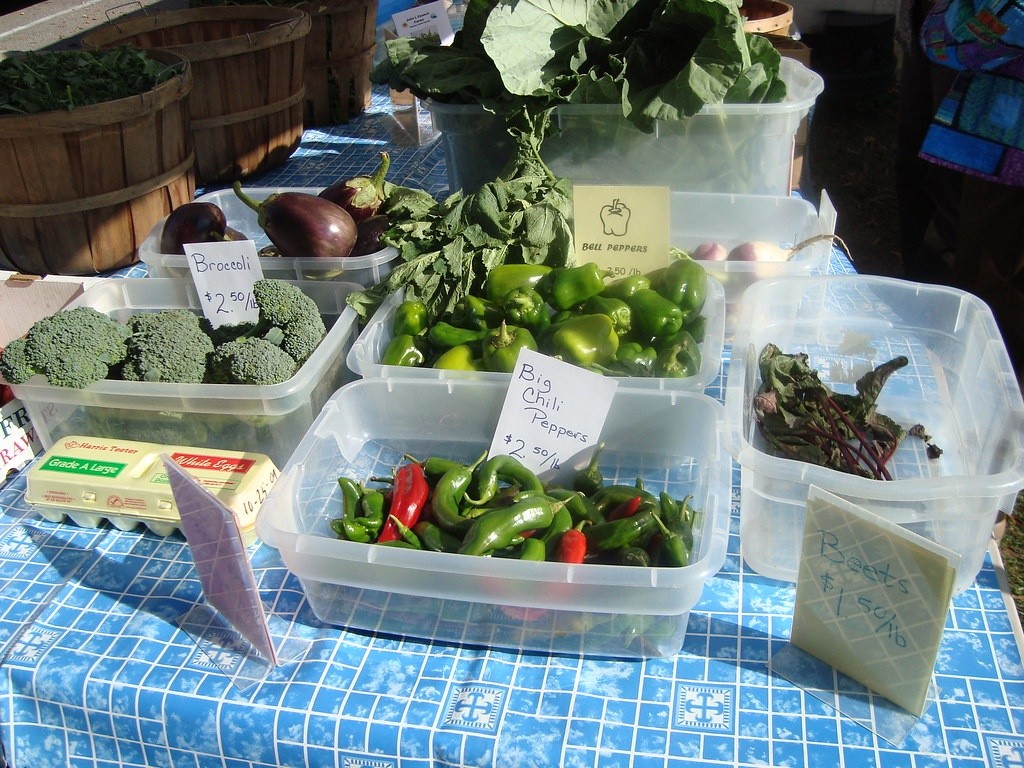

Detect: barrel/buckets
235,0,378,128
80,3,310,183
1,50,194,274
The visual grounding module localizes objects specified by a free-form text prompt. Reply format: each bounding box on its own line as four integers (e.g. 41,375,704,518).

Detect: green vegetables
0,44,185,116
368,0,792,197
748,342,944,483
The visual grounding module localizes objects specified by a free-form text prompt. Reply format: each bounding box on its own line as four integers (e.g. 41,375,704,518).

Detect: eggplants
159,152,396,286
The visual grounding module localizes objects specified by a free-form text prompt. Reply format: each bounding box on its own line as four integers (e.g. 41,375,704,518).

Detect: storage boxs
137,186,404,289
347,275,726,396
0,270,120,491
250,374,735,661
8,272,361,474
668,189,834,349
417,54,825,203
384,27,413,106
720,273,1024,596
25,433,279,555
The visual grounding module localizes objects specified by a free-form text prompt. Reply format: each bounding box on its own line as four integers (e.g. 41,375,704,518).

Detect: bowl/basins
344,270,726,394
572,194,824,345
722,275,1024,592
2,278,365,466
136,187,416,284
256,378,733,661
418,57,826,194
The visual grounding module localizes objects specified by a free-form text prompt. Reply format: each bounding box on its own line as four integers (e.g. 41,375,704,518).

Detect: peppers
379,257,710,379
324,441,697,655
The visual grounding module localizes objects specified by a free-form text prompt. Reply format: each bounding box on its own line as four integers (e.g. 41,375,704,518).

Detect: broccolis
0,278,329,456
